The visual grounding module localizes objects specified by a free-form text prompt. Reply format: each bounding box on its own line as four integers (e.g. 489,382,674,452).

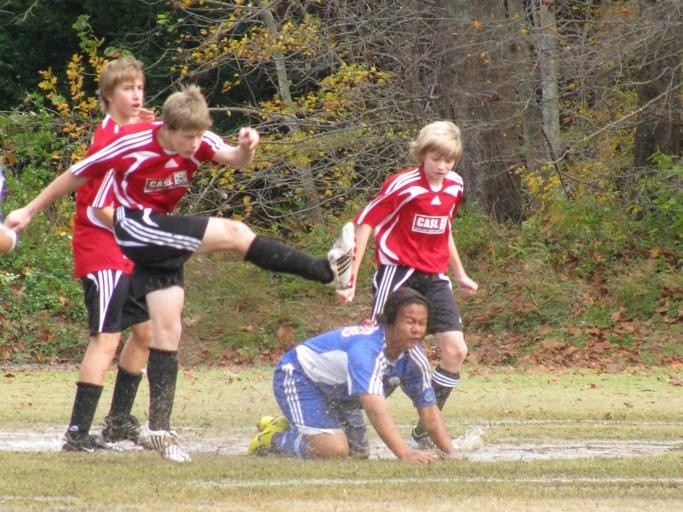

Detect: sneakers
256,415,276,433
322,221,355,290
410,428,435,449
247,415,291,456
137,421,192,464
60,432,106,453
102,414,141,443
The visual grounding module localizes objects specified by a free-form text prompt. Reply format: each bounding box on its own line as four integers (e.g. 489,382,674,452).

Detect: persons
331,119,479,455
59,54,155,456
1,77,357,469
243,283,465,467
